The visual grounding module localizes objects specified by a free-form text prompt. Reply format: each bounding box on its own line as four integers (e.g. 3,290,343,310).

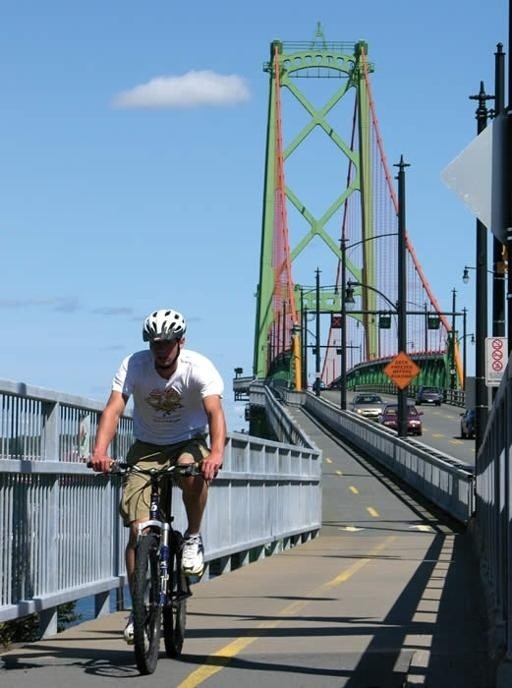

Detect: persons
87,307,228,642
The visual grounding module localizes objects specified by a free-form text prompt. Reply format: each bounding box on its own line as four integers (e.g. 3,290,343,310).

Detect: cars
378,404,424,435
416,386,445,405
313,381,326,391
458,406,477,439
348,393,387,422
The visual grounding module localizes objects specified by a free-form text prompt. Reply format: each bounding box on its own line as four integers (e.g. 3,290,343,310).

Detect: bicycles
83,457,224,676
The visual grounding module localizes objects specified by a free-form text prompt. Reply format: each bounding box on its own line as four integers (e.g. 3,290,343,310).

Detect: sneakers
180,531,205,576
125,604,136,642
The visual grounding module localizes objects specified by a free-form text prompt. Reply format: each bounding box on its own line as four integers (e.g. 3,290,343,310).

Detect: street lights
289,266,322,398
456,334,476,346
464,266,510,300
344,154,411,439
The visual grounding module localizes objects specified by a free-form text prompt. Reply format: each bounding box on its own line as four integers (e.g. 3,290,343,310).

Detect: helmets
143,311,187,342
